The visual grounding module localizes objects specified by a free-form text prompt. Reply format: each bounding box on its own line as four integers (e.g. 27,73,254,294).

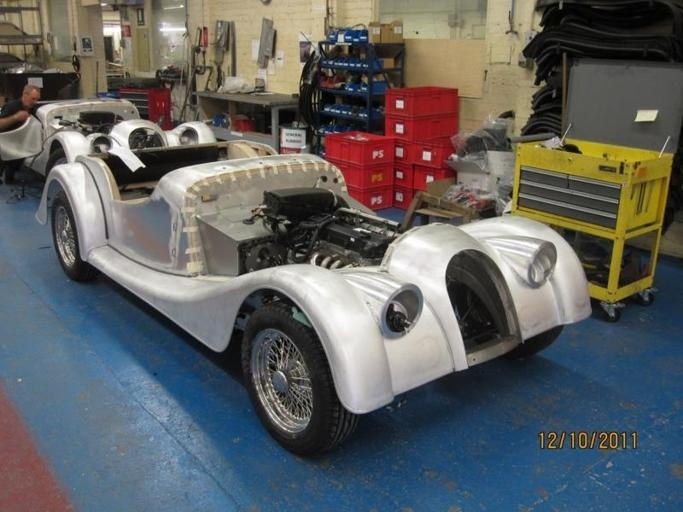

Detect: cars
35,118,593,461
1,93,217,182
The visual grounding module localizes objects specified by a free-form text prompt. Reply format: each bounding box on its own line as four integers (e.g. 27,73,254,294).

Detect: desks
192,90,300,155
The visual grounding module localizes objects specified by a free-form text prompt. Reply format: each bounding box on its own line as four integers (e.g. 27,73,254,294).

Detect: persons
0,85,41,186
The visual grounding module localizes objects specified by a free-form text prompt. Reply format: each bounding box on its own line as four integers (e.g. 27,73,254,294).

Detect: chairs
91,143,219,200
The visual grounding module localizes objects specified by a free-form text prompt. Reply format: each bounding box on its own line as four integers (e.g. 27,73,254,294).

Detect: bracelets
14,114,19,121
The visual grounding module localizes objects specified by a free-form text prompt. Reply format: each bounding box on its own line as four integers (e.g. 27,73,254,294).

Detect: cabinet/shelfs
0,0,44,68
310,41,404,158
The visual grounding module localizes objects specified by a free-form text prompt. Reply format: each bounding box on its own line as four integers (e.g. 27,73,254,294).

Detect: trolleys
501,137,669,321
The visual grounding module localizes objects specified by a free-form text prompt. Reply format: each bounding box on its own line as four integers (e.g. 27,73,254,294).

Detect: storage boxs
325,131,394,211
413,137,455,194
386,86,461,210
